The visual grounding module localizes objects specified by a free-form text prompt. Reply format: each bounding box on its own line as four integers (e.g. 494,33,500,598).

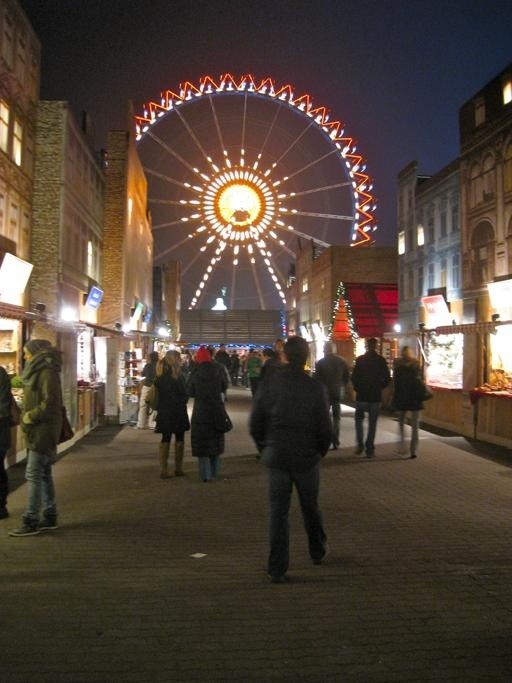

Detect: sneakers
9,515,57,537
354,448,373,457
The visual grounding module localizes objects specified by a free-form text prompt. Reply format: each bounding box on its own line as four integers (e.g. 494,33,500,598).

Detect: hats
196,347,211,363
26,340,50,355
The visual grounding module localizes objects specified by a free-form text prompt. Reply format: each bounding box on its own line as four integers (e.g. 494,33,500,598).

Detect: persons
309,339,349,450
140,343,305,485
349,338,391,461
388,344,430,461
8,340,63,536
0,366,22,518
246,336,334,584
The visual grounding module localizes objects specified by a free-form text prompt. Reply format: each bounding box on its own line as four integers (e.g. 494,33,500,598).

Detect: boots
160,441,184,479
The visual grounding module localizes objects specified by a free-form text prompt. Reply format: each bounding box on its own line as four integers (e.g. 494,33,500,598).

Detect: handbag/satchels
57,407,74,444
146,385,159,410
419,384,433,401
214,408,232,432
